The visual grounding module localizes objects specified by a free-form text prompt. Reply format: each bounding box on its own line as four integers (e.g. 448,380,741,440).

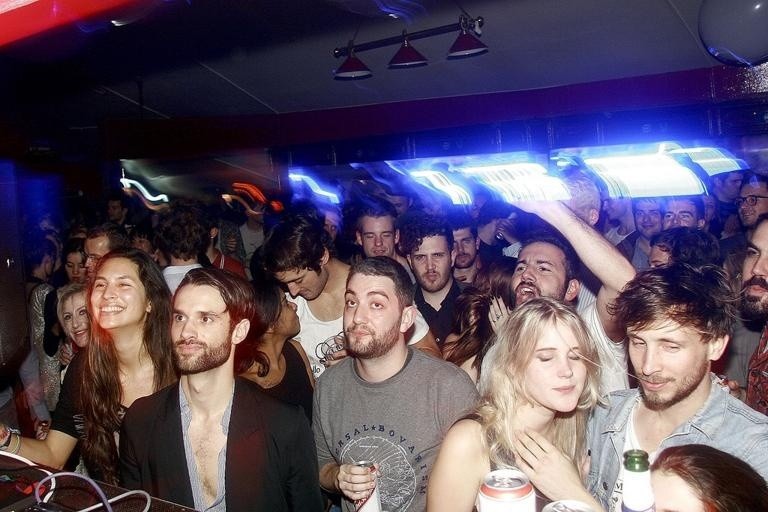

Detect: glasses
733,195,767,206
79,250,102,261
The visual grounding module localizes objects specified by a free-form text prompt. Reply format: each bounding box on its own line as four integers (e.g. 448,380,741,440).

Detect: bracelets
1,424,7,438
1,428,12,451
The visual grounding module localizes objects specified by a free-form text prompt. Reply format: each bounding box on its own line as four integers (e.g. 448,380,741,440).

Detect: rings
493,318,498,322
497,314,502,320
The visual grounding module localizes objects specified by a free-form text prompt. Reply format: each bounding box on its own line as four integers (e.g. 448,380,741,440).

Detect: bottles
621,449,657,509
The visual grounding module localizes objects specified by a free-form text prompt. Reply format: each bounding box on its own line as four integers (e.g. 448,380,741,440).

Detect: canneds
477,468,537,512
354,461,382,511
497,211,518,240
35,420,48,440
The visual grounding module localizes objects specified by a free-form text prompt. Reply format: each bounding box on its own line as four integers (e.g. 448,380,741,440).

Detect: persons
118,267,322,511
650,444,767,511
586,261,768,510
1,165,767,485
426,294,616,511
312,254,487,511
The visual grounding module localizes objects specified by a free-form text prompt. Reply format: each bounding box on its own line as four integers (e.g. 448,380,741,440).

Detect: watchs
8,427,21,454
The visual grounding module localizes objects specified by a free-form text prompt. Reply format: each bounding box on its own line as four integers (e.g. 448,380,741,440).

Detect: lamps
331,1,488,82
